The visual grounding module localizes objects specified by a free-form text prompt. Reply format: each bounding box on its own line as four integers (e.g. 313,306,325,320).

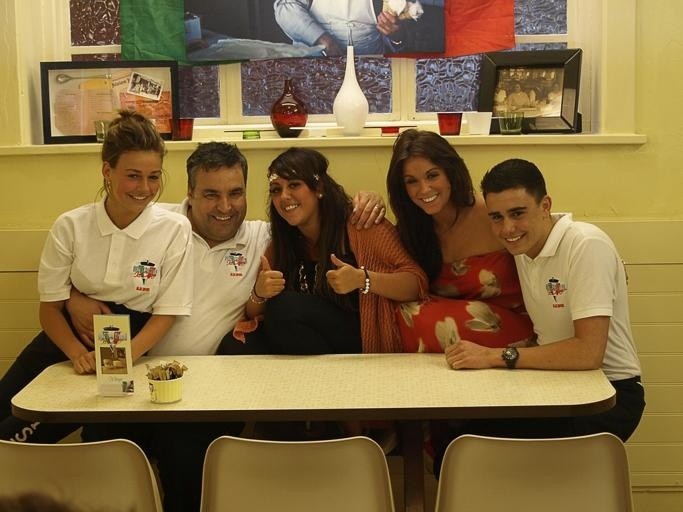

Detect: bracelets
253,285,269,301
249,293,264,305
358,264,371,294
390,38,404,46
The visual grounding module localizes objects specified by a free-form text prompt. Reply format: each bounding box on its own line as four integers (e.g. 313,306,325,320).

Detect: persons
65,140,386,512
272,1,408,56
130,76,141,93
386,129,534,512
434,158,644,480
493,77,562,108
232,146,431,511
1,109,193,442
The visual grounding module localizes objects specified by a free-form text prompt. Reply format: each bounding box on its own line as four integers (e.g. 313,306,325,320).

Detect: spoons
56,73,113,84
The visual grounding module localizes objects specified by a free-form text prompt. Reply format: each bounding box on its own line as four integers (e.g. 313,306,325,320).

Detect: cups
147,377,182,404
467,111,493,135
499,112,524,134
169,118,193,140
437,112,462,135
185,17,203,39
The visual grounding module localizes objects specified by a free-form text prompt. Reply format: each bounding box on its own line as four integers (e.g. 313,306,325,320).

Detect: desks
8,349,619,512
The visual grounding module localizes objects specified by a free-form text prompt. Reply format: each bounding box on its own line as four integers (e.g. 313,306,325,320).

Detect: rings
375,205,381,210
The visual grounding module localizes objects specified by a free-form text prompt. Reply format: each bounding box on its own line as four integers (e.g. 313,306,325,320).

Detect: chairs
430,432,634,511
197,431,396,512
0,436,166,512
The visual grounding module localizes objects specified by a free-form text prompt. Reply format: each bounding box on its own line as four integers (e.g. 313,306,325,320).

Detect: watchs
501,346,519,370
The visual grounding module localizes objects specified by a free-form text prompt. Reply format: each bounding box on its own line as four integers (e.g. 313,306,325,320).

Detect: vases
329,46,369,137
269,77,309,138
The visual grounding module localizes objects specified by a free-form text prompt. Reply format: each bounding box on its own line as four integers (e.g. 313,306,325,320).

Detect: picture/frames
475,47,584,135
38,58,182,146
